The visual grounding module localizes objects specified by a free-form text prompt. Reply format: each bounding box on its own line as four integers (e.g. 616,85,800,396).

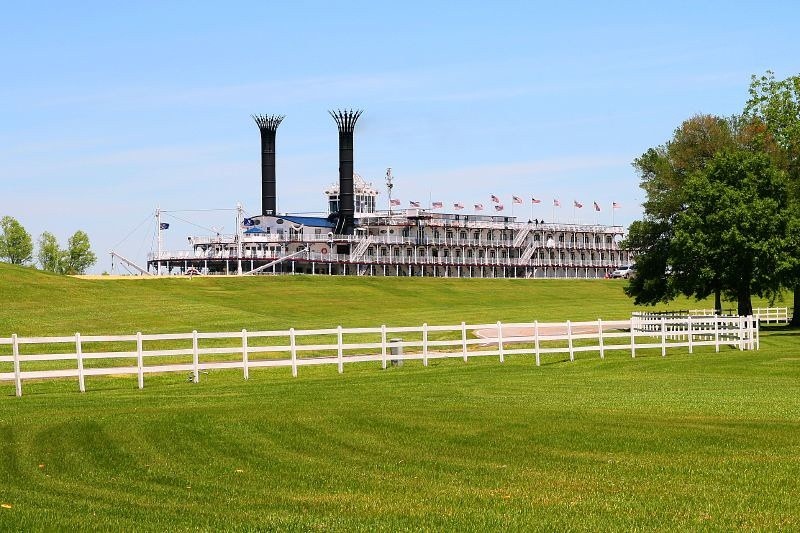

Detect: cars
612,265,637,278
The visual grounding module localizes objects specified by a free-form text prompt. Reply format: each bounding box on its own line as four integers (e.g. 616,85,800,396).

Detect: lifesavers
321,248,327,254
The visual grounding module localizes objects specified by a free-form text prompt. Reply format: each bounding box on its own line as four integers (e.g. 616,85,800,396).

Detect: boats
109,108,636,277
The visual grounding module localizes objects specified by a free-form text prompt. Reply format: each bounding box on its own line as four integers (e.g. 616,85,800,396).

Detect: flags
473,202,484,211
573,200,582,208
532,198,541,203
613,201,621,208
552,199,561,206
491,193,500,203
390,199,400,206
409,200,421,208
243,218,255,226
160,222,169,230
593,201,600,211
512,193,522,204
452,200,464,210
494,204,503,212
432,201,443,210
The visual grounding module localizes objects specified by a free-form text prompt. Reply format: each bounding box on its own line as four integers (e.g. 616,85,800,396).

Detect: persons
604,271,612,279
